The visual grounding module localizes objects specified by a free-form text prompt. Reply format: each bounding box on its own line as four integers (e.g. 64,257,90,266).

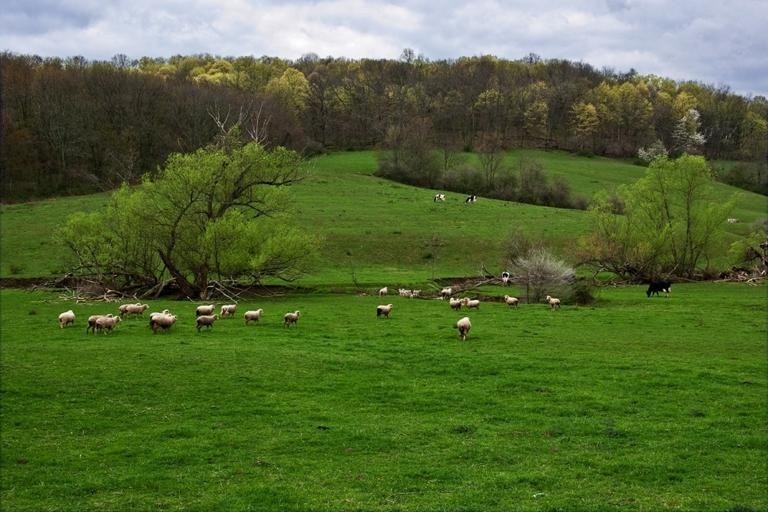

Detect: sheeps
377,303,393,319
283,310,300,329
379,286,388,296
398,288,422,300
546,295,561,312
456,316,471,341
441,288,480,310
504,294,519,308
58,302,264,336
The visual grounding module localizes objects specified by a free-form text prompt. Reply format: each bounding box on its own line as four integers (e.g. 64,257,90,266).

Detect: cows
646,280,672,297
501,272,511,286
466,193,477,204
434,194,445,202
